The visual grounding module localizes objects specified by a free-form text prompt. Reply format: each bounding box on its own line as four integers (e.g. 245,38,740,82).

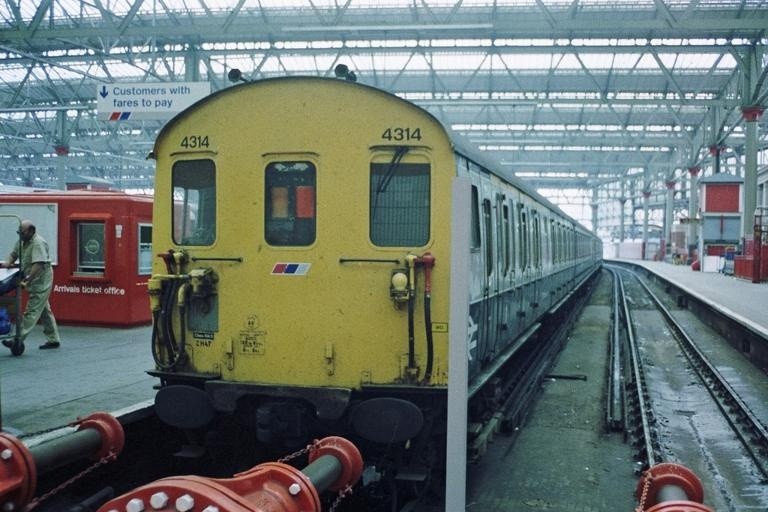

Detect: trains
0,66,608,511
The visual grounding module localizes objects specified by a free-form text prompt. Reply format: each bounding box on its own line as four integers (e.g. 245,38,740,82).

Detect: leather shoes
39,341,60,350
3,339,15,348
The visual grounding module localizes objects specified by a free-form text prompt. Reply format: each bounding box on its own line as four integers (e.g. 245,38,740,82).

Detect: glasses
16,230,26,235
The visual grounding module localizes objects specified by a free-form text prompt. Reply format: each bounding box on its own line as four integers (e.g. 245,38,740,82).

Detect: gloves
20,275,33,289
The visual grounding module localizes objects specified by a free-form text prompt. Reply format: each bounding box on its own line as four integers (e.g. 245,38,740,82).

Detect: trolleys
0,213,29,361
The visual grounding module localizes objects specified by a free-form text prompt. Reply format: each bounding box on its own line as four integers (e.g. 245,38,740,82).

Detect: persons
2,220,60,350
